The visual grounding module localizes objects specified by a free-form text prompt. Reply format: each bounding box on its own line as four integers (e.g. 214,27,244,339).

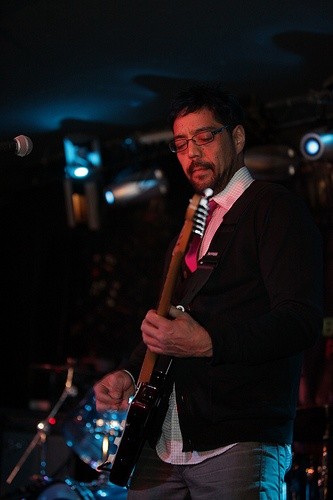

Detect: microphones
0,135,33,157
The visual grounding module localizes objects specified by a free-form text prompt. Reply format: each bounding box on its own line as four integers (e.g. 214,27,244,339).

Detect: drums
39,383,138,500
294,406,333,446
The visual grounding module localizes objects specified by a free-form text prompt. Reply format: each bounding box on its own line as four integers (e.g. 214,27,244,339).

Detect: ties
180,200,218,299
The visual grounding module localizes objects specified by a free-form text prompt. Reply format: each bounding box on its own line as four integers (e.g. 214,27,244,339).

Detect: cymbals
39,358,114,371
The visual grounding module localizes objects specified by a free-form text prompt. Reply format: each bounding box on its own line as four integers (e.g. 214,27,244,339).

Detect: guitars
108,193,210,487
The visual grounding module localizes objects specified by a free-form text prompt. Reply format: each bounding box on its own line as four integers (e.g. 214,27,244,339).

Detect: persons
93,87,323,500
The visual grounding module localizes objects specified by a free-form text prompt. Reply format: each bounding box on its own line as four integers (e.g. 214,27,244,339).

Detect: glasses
169,124,231,153
